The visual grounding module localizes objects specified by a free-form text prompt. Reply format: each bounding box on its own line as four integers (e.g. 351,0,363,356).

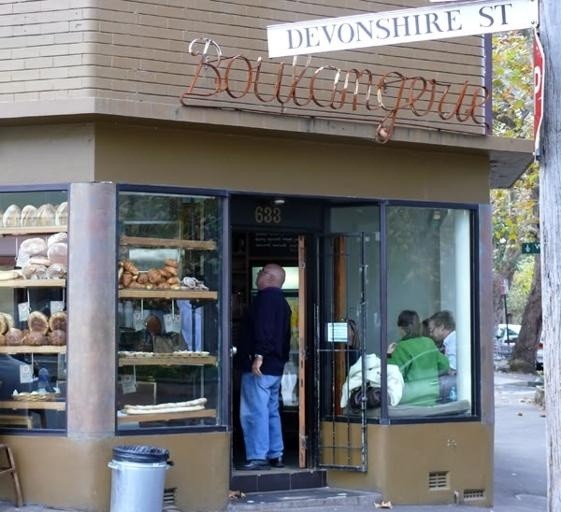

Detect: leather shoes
270,456,285,467
238,459,270,471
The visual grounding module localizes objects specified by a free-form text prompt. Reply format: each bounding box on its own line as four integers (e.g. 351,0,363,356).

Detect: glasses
258,268,269,275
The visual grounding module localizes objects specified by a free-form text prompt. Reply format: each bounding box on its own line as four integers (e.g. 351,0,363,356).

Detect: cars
496,323,544,369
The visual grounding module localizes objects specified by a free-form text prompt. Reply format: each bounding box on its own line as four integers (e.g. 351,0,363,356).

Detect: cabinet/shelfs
118,234,218,422
0,226,68,410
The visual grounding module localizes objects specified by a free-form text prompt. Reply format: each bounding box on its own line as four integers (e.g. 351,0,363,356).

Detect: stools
0,444,23,507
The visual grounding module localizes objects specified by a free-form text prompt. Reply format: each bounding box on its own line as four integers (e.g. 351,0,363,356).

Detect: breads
1,201,181,346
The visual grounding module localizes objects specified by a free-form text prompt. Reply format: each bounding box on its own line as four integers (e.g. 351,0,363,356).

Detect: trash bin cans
107,445,175,512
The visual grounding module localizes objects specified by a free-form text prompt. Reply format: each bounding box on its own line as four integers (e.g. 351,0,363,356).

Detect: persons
236,264,292,470
0,351,32,428
344,309,456,407
